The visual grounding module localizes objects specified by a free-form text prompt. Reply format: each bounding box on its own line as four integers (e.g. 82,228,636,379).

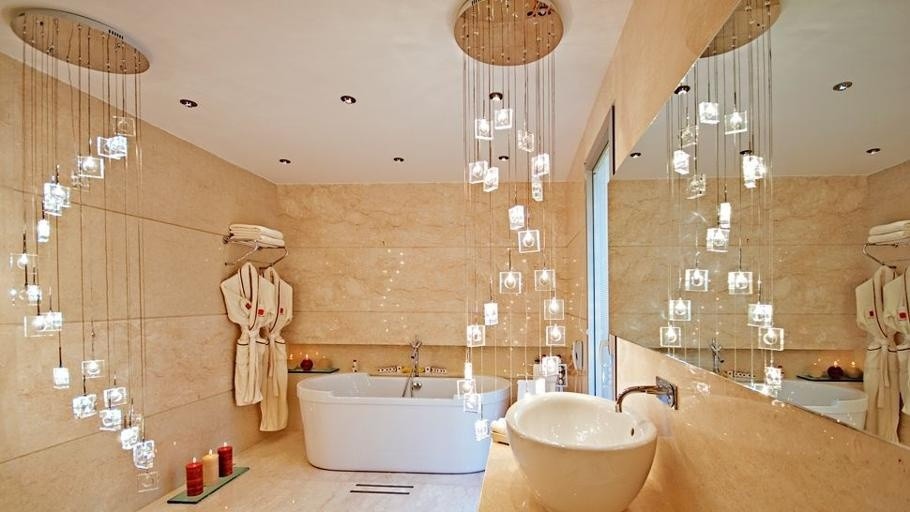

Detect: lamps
453,0,567,440
7,11,160,474
658,1,784,400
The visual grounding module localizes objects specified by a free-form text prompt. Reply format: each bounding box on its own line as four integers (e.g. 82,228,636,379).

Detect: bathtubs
296,372,510,474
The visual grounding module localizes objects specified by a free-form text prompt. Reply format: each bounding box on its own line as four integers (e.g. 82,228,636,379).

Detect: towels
228,222,284,248
867,219,910,243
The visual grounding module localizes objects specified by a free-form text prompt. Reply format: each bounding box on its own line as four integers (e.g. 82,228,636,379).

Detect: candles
288,353,333,370
808,361,861,378
185,441,232,496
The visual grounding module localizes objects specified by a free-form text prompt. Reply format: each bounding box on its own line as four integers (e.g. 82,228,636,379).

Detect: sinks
505,393,658,512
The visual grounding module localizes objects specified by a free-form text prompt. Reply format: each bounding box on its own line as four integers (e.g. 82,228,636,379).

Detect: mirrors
608,2,909,451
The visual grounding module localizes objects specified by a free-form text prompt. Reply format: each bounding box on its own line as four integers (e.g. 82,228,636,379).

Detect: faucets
614,375,677,414
409,333,423,376
